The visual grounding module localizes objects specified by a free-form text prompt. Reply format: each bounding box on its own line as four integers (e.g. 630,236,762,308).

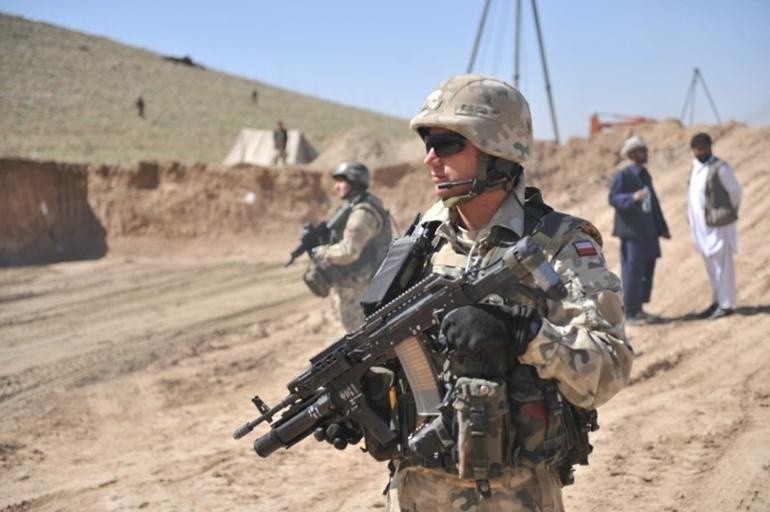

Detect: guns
284,206,351,268
233,237,568,457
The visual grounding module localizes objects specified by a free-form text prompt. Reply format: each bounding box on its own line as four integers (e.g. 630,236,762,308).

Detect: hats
691,134,711,147
620,134,648,157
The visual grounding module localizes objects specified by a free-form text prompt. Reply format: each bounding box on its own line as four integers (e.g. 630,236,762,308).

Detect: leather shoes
629,312,659,324
701,302,732,318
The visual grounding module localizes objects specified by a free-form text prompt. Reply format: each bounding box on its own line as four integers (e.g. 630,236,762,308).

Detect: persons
136,93,145,116
313,75,634,512
271,119,287,165
608,135,672,324
314,159,393,334
687,134,743,319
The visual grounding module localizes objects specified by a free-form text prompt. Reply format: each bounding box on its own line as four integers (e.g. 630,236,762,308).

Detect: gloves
314,421,363,450
441,305,514,352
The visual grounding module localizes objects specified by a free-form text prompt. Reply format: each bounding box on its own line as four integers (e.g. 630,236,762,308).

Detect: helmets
332,160,369,189
409,74,533,166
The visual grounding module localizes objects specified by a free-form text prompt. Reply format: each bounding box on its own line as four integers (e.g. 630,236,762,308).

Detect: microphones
438,178,473,189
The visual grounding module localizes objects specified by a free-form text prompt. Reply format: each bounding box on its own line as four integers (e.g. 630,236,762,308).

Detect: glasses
421,133,466,157
